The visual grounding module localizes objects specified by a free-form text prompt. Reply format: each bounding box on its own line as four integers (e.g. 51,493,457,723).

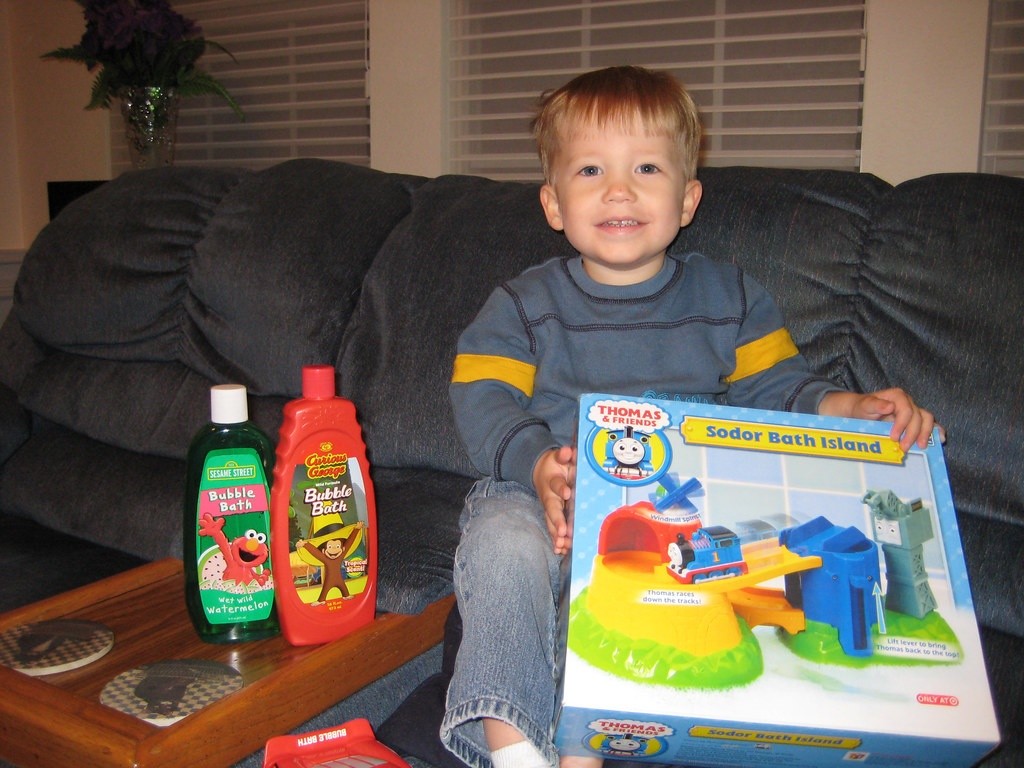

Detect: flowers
37,0,245,166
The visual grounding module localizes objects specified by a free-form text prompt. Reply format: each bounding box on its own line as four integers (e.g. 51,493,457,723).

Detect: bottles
270,363,378,647
184,383,281,644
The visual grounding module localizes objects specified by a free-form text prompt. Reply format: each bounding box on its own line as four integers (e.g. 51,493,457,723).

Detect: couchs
0,160,1024,768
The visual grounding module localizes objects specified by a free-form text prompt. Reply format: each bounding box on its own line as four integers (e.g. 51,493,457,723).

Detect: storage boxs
551,392,1001,768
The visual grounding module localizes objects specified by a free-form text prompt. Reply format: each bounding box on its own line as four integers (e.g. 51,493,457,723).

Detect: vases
118,85,181,166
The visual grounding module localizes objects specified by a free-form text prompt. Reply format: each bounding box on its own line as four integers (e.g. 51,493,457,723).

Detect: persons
437,65,946,768
